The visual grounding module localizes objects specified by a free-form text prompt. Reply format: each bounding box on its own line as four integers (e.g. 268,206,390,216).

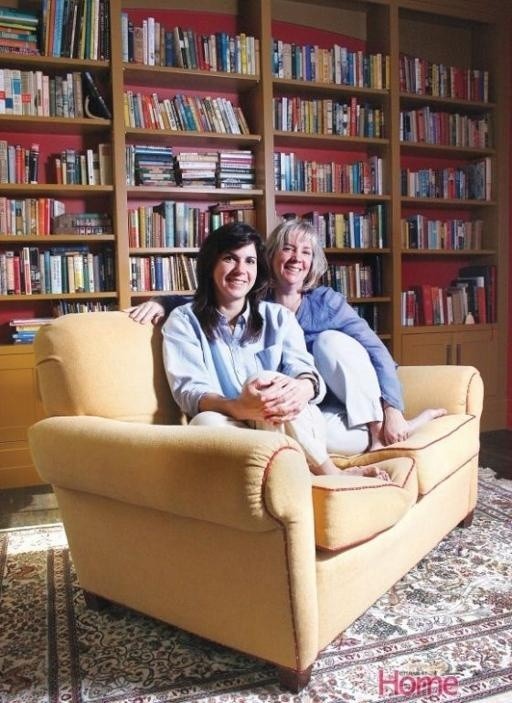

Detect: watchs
307,377,321,401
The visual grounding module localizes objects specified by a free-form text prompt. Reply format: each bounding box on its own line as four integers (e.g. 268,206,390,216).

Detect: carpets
0,467,512,703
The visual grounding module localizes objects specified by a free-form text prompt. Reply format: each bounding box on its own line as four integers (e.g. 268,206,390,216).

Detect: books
120,12,258,292
401,265,498,328
397,55,493,249
272,38,390,334
0,0,117,345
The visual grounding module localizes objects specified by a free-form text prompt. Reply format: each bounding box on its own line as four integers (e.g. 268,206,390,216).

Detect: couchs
29,312,484,693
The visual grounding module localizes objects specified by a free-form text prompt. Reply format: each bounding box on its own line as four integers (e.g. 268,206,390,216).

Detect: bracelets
383,404,396,410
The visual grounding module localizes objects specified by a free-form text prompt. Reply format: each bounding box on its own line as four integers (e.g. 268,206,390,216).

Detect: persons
159,222,392,482
122,219,447,456
